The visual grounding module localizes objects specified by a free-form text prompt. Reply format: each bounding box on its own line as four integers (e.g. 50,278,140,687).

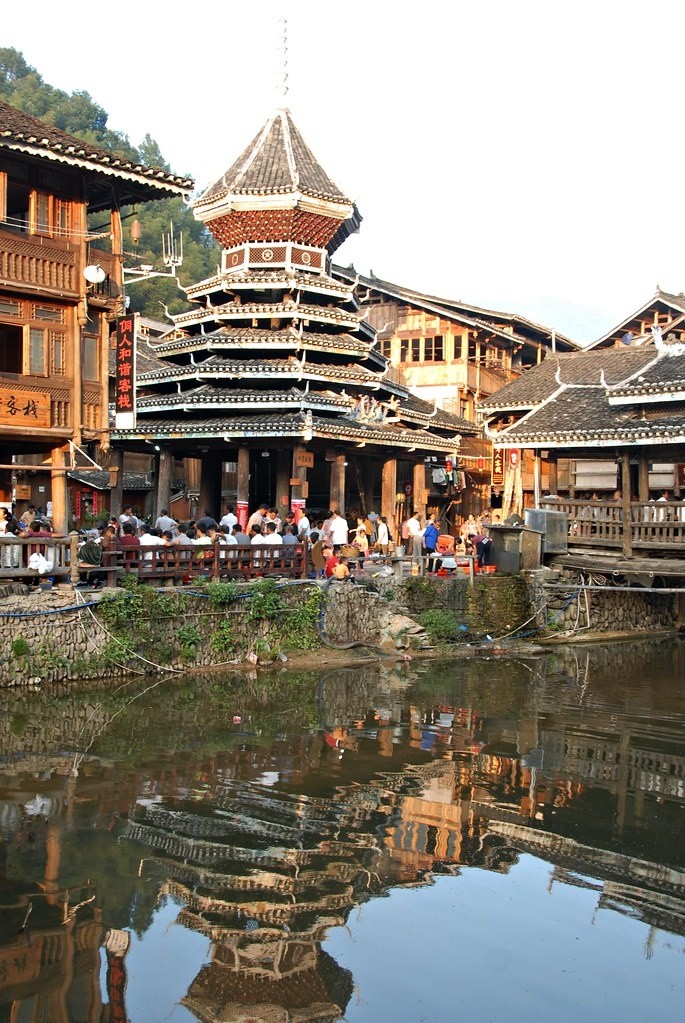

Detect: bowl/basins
438,569,450,576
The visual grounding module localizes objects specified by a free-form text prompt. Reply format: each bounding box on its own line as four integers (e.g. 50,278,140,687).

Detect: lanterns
478,457,484,472
446,460,452,472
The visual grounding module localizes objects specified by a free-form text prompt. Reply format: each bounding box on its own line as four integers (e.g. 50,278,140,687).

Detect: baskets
341,544,359,558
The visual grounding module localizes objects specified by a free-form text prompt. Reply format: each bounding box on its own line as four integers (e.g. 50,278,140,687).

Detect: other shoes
431,572,438,577
452,570,458,575
475,572,482,576
483,571,490,575
360,568,364,572
376,560,383,565
427,571,434,575
73,580,88,587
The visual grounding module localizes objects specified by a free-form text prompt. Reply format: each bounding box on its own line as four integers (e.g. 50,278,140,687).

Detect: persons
541,489,685,535
496,415,514,433
80,501,504,586
0,496,88,590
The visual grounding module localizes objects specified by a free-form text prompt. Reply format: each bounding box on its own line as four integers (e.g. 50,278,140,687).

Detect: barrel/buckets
395,545,406,557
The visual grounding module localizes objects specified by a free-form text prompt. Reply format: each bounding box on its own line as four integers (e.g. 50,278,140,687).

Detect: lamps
83,265,106,292
